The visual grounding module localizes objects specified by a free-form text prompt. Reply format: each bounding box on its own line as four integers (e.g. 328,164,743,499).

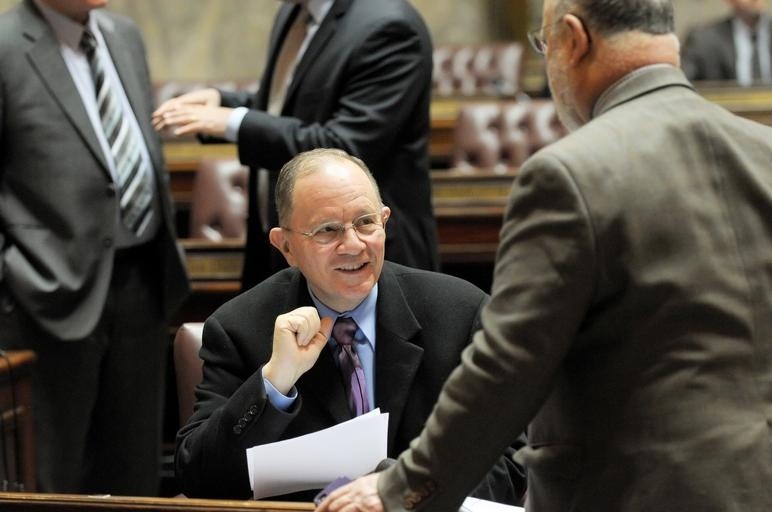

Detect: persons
0,0,194,512
149,0,446,292
679,1,772,84
312,1,772,512
172,147,527,512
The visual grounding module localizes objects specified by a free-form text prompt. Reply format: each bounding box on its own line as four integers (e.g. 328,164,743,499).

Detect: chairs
149,38,573,433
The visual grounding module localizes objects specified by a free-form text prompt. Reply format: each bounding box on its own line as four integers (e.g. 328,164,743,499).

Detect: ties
749,26,763,83
266,7,313,116
330,316,369,418
78,28,156,238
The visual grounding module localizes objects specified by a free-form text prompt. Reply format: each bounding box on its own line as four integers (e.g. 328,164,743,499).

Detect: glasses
526,20,558,55
285,212,383,244
